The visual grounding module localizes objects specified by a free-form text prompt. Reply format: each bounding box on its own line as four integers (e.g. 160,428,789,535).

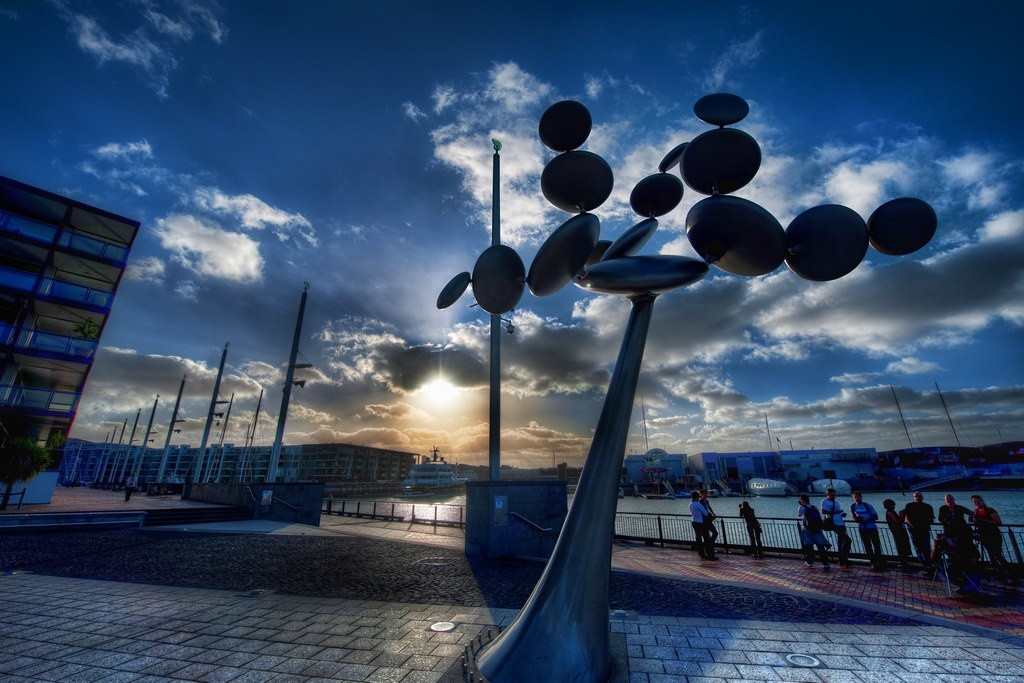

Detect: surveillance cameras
507,325,515,335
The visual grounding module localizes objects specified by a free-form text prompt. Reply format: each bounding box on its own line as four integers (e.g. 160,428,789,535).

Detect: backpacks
802,504,824,532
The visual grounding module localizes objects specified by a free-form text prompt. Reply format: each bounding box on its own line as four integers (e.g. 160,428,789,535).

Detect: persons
904,491,936,575
739,501,764,559
820,488,852,565
124,473,137,502
797,495,832,570
850,491,885,569
930,533,986,595
971,495,1018,583
938,495,973,542
883,499,907,573
688,489,720,560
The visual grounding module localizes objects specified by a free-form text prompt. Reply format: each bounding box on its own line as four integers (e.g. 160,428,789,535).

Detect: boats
399,445,470,490
640,480,742,500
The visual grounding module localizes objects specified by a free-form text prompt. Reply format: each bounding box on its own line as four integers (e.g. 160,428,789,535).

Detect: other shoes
751,550,757,554
700,554,707,559
901,562,915,570
838,559,851,566
924,564,934,572
804,561,813,567
706,554,716,560
758,550,762,554
822,563,830,567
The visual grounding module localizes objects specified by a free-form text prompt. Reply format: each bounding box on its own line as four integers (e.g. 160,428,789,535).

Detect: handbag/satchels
821,517,833,531
972,529,982,541
